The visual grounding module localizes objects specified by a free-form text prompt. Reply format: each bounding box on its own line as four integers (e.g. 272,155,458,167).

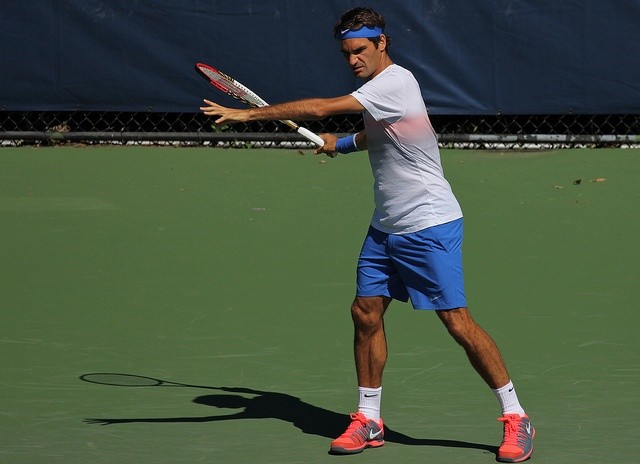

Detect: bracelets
333,132,359,156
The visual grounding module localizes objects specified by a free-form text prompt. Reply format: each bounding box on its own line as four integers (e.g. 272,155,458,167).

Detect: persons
199,7,537,460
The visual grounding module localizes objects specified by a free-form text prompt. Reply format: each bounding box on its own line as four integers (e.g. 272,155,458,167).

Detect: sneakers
497,413,536,463
331,412,384,455
